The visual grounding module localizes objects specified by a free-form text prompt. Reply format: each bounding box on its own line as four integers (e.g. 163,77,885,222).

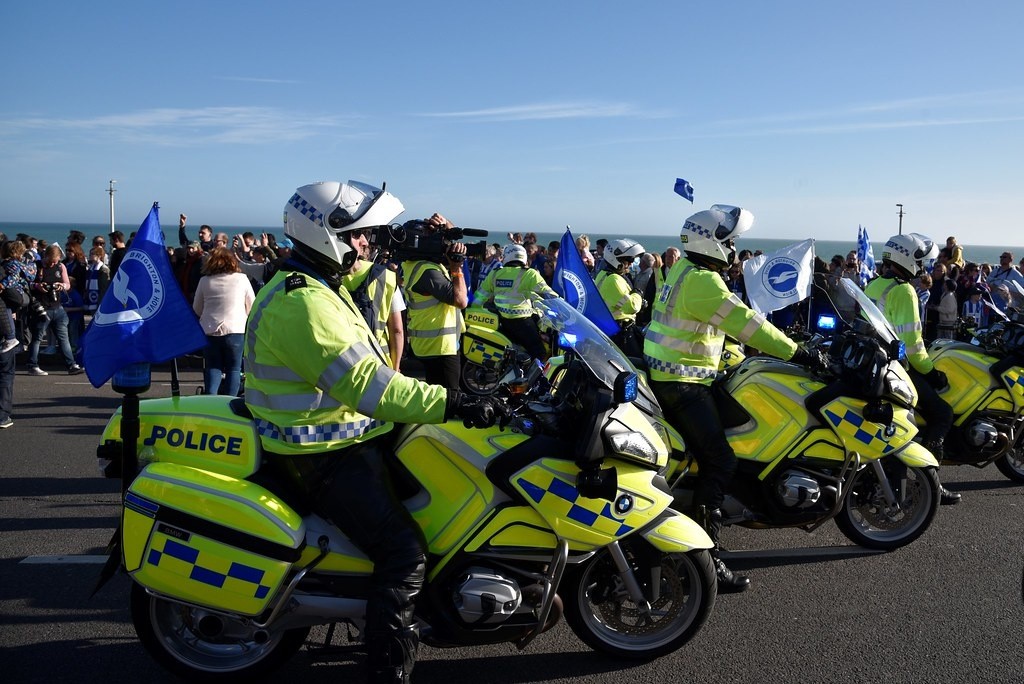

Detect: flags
79,202,208,389
542,231,619,351
739,238,815,318
673,177,694,204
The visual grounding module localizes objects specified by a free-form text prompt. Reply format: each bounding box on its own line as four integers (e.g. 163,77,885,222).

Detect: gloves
442,387,499,429
925,366,947,390
789,347,827,375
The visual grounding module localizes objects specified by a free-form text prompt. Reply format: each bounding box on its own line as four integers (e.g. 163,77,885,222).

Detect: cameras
848,263,854,268
44,283,59,302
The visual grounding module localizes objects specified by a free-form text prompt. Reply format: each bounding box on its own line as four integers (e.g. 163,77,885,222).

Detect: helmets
603,239,645,268
502,245,527,268
882,234,941,278
680,204,756,266
283,180,406,275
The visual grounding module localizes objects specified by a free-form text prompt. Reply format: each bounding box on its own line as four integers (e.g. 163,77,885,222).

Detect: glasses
350,227,370,241
1000,255,1010,259
213,239,224,243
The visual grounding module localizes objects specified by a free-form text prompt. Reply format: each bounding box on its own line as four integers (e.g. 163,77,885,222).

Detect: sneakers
28,366,48,376
0,415,13,428
67,365,85,375
0,338,20,354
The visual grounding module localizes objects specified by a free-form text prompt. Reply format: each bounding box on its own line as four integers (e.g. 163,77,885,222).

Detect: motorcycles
94,296,940,684
902,279,1024,482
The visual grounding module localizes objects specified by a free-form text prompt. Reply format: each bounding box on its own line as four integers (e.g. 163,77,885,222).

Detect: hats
276,238,293,250
970,288,985,295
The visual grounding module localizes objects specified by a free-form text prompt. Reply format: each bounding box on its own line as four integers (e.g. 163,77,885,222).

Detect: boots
365,615,421,684
694,503,751,591
924,439,962,504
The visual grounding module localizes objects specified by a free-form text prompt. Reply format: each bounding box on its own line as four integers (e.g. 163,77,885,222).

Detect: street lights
106,179,117,233
896,203,907,235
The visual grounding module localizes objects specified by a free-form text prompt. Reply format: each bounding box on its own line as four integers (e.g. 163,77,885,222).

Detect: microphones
463,227,488,237
357,255,364,260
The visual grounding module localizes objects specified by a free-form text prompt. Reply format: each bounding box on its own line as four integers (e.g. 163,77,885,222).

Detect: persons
862,232,961,506
246,179,512,684
641,204,829,596
0,214,1024,429
